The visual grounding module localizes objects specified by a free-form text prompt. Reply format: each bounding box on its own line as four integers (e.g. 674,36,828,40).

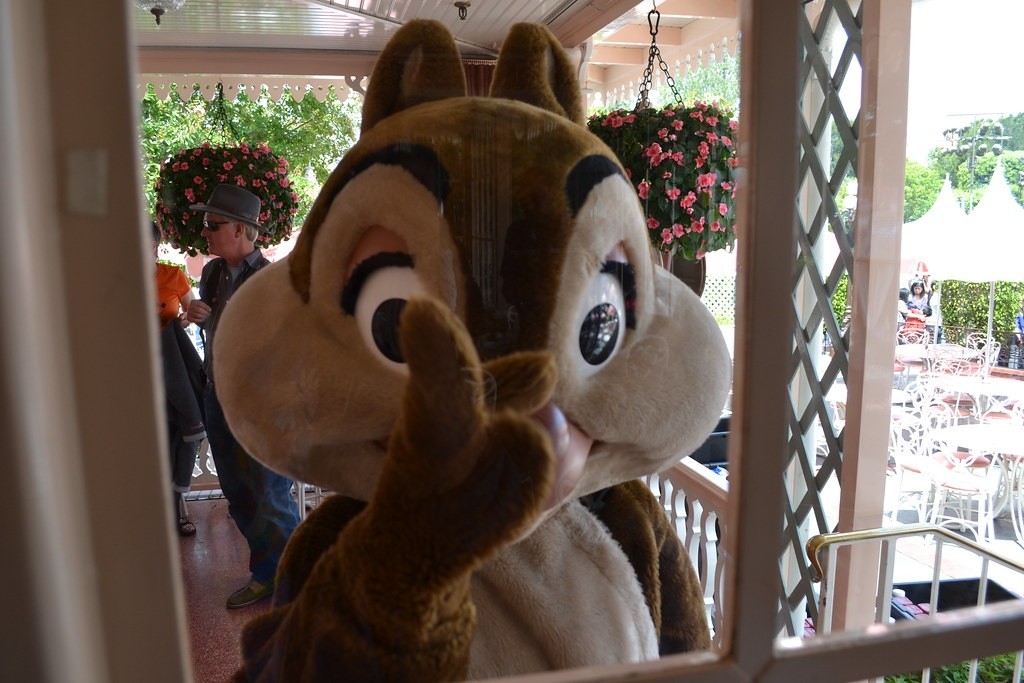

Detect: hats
188,184,272,234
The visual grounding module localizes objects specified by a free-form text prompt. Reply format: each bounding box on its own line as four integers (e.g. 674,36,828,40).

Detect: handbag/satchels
904,317,925,336
923,306,933,316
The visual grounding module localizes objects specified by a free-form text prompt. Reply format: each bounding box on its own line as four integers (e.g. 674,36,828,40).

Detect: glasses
203,219,229,232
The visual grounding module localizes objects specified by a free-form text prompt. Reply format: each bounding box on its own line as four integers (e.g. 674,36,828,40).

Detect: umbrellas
843,161,967,318
919,160,1024,385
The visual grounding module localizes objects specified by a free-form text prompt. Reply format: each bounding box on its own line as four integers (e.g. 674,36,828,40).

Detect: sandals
176,516,197,536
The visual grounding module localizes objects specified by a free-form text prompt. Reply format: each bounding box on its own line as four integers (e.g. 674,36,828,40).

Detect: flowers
155,142,298,257
587,100,741,259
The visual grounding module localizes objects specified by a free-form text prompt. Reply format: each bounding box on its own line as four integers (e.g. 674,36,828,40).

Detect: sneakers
227,579,268,608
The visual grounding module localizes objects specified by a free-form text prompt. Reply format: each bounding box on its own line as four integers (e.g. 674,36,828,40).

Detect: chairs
804,328,1024,547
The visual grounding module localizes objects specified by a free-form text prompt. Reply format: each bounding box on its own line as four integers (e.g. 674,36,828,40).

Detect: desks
929,423,1024,548
931,376,1024,425
896,344,978,382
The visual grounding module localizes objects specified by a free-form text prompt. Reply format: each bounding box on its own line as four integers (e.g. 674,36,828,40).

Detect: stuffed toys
208,21,730,683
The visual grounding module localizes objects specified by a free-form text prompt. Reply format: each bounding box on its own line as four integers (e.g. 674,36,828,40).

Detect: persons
150,222,196,538
897,281,942,344
1014,299,1024,369
187,186,304,612
